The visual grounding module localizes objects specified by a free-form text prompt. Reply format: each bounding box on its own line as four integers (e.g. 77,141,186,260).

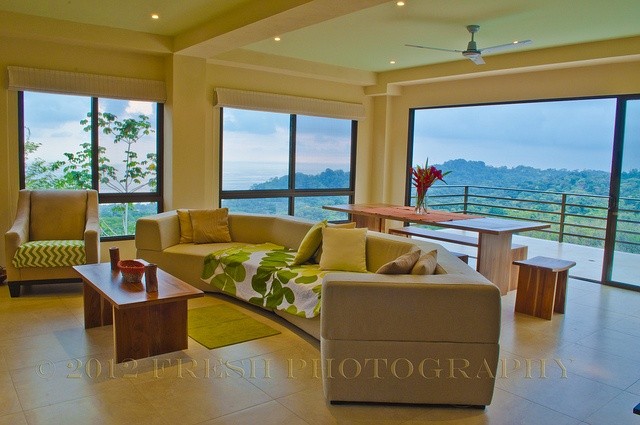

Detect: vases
414,189,430,214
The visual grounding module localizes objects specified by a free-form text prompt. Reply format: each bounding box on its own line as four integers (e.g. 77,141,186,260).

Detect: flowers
409,156,452,213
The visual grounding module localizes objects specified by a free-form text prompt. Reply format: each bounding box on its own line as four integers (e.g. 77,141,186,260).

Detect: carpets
188,303,281,351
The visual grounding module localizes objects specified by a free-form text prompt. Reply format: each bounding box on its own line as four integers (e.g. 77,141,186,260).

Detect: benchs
388,227,527,290
452,250,468,263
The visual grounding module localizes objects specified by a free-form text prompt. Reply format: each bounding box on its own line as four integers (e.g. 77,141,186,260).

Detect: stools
513,255,576,320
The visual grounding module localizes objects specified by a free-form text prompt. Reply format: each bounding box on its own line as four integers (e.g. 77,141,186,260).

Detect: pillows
374,249,420,274
176,208,193,244
188,208,232,244
313,222,356,262
319,226,367,272
295,218,328,265
410,249,437,274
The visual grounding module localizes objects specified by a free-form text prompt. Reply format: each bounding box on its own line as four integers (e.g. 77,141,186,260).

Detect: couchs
5,189,101,297
135,202,501,405
